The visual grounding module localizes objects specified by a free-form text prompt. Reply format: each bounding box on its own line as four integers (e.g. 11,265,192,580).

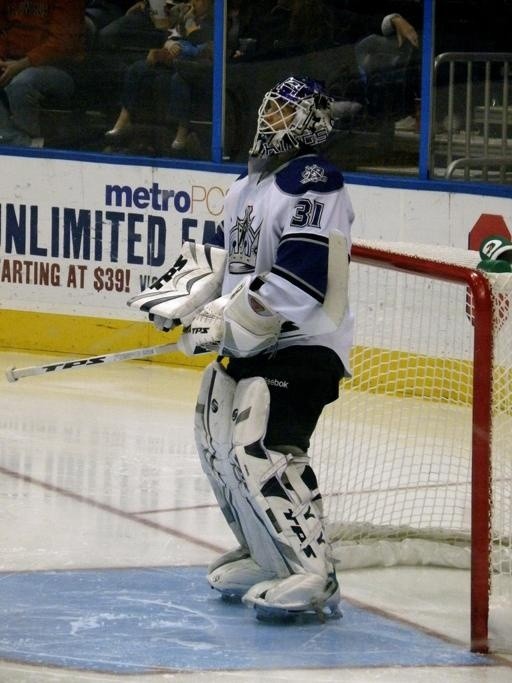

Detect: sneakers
171,134,192,151
105,121,134,138
395,115,417,130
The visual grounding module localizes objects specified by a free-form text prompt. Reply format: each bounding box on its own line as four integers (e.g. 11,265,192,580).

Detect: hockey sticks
4,228,349,381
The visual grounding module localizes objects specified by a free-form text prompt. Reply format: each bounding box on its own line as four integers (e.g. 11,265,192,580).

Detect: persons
1,0,419,169
125,76,355,612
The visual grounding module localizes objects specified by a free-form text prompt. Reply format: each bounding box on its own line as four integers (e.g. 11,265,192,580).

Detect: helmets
249,75,337,158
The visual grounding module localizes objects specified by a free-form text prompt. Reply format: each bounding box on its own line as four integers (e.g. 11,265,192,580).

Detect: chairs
1,2,392,165
